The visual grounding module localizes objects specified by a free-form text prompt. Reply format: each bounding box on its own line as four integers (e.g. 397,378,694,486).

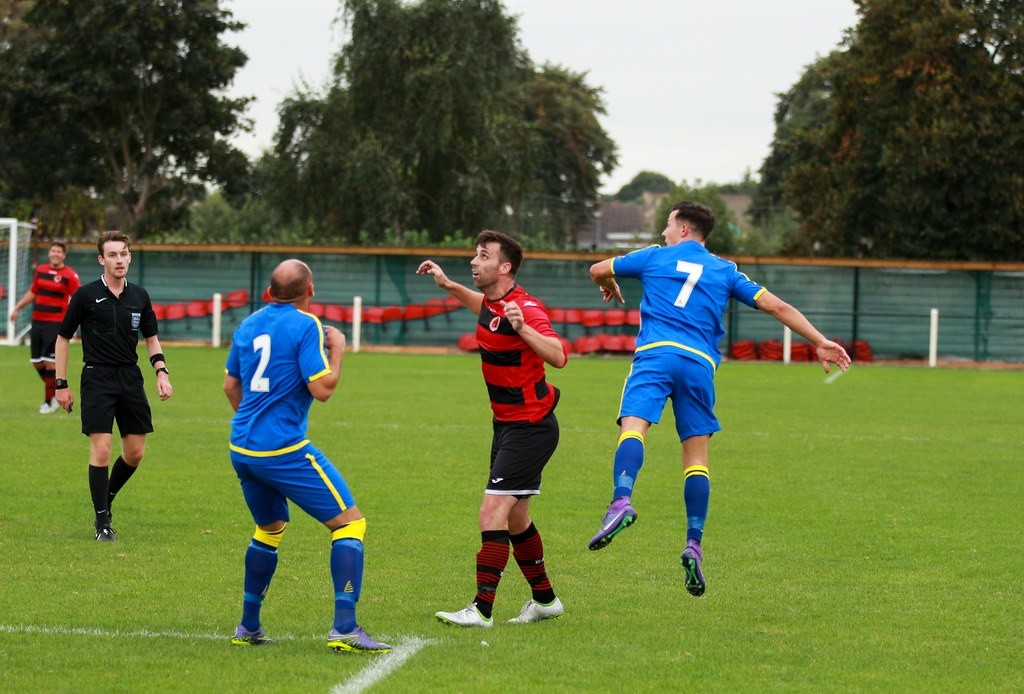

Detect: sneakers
51,396,60,413
230,624,276,647
435,602,494,628
40,402,51,414
326,623,393,655
507,596,564,624
93,510,116,542
588,496,638,551
677,538,706,597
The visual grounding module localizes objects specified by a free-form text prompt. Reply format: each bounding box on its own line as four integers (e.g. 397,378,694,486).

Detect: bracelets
149,354,165,367
55,385,68,389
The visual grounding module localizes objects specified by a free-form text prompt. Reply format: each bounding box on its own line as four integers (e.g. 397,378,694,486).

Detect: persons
223,259,392,654
55,230,173,542
10,241,80,415
415,231,569,630
589,200,851,596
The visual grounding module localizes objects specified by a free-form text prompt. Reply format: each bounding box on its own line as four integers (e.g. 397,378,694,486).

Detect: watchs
156,367,169,376
56,379,67,386
